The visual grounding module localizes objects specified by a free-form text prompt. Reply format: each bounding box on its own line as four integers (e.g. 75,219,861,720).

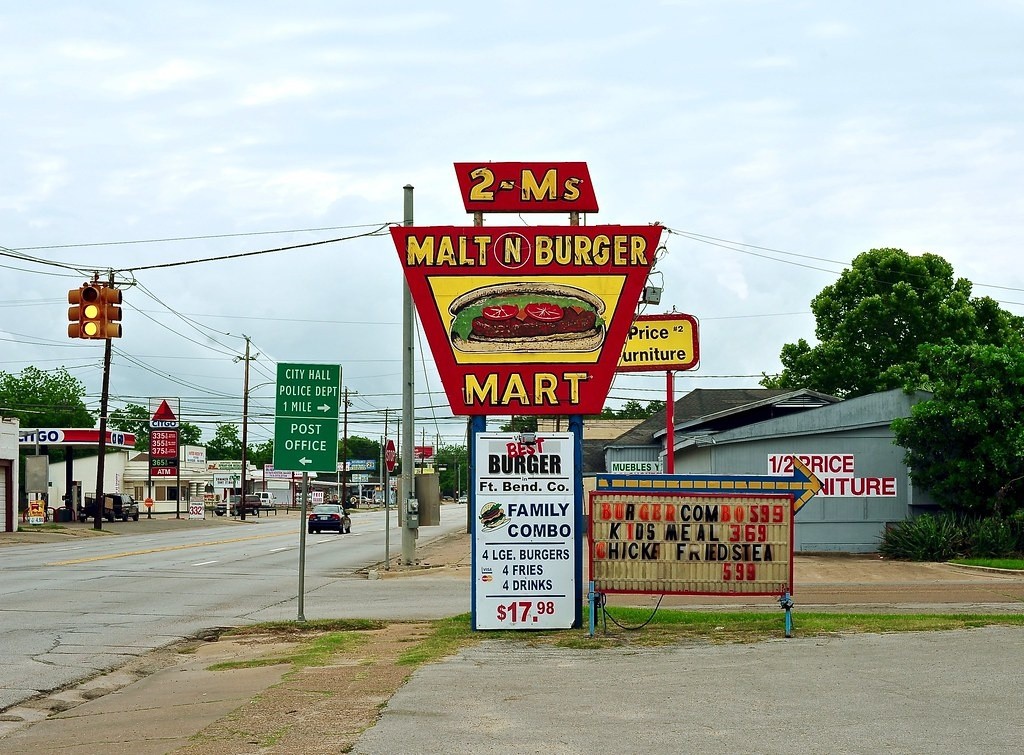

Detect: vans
254,492,277,508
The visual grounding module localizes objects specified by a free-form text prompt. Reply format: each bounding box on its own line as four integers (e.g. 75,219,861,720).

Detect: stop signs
384,440,396,472
145,498,153,507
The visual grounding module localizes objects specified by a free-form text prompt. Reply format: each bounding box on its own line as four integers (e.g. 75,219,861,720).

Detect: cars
458,495,468,505
307,504,352,534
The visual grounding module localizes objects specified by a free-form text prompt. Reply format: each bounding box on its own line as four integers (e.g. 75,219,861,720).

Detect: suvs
215,495,262,516
346,495,357,509
355,496,373,504
105,493,140,522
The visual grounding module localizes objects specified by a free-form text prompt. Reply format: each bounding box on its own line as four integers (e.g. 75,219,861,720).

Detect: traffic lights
67,286,123,340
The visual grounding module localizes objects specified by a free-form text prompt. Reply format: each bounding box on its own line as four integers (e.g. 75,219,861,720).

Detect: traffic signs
272,362,343,474
